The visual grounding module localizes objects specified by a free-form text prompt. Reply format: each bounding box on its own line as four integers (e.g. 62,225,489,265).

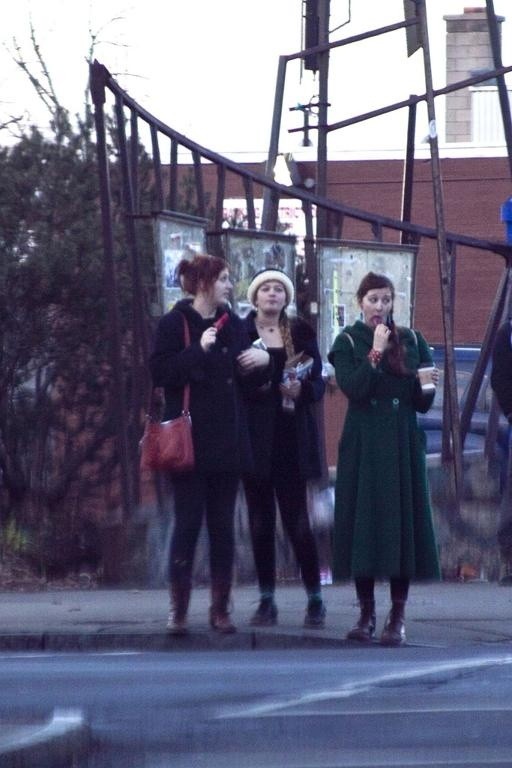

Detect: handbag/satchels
138,409,195,474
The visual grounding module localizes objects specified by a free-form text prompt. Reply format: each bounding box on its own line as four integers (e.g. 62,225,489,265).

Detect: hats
247,268,295,309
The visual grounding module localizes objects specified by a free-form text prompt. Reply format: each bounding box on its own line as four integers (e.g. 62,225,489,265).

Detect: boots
209,577,236,634
166,576,189,633
381,600,408,646
348,600,376,642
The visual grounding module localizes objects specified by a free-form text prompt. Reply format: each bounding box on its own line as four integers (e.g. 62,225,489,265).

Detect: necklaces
257,319,278,332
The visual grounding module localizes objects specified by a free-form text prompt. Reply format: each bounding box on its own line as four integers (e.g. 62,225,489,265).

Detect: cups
416,363,435,391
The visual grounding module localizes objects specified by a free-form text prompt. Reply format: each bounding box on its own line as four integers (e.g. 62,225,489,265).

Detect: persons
147,254,275,634
489,320,512,586
235,267,328,631
327,271,439,646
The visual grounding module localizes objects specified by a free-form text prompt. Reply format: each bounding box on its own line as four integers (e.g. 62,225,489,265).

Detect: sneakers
304,599,326,628
248,597,279,626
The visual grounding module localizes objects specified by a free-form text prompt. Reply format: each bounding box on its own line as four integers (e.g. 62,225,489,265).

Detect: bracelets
367,348,383,364
